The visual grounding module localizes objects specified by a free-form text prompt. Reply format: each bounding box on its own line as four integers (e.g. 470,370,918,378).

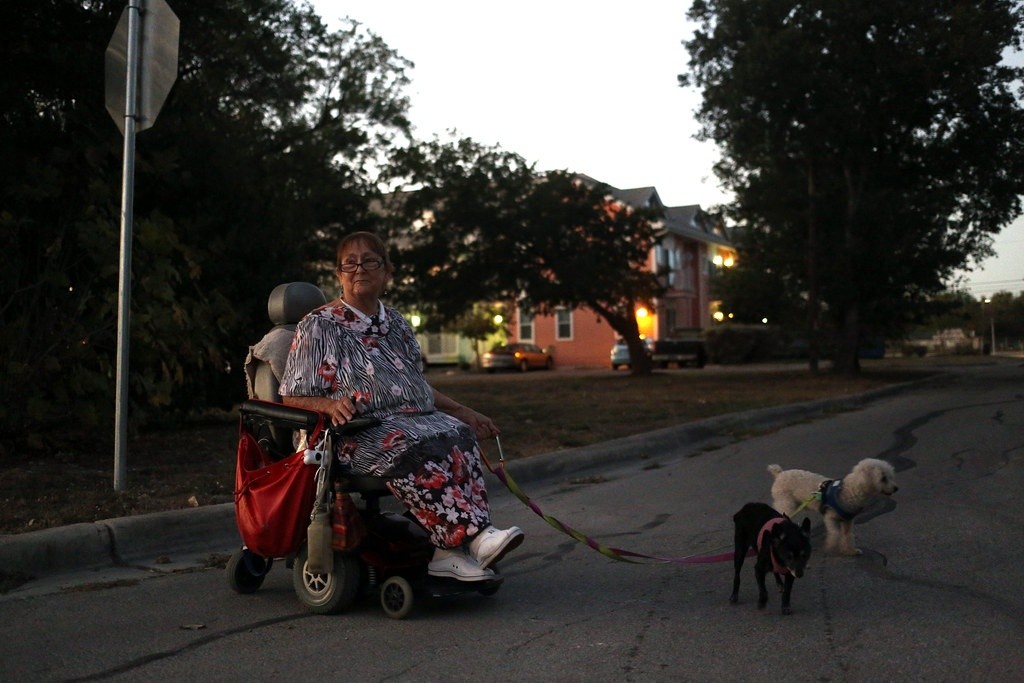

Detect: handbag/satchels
233,394,328,560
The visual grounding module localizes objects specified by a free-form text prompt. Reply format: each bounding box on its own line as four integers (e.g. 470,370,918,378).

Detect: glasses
338,260,386,273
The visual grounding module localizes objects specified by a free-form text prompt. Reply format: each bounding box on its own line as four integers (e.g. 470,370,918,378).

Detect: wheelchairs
226,280,500,619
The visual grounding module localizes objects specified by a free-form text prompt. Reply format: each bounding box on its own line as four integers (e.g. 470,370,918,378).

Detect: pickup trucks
654,327,712,365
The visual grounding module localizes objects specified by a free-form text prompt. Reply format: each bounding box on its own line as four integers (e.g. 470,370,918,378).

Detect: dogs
767,458,898,557
729,502,811,616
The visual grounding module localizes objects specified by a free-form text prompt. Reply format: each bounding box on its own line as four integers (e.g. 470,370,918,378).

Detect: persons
278,230,525,581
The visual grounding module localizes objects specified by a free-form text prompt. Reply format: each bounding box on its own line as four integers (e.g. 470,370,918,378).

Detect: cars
482,341,553,372
610,335,656,368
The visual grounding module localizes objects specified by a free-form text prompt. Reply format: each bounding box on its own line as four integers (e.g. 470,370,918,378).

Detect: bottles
307,511,334,574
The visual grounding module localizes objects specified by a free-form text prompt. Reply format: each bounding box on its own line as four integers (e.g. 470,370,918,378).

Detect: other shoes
469,526,526,570
426,551,496,581
